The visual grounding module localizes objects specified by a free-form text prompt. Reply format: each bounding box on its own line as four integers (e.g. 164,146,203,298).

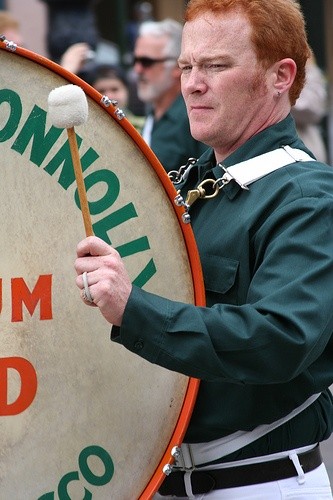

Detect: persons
290,44,328,163
74,0,333,500
0,0,212,179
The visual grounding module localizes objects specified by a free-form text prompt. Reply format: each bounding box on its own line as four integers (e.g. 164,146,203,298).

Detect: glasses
133,57,165,66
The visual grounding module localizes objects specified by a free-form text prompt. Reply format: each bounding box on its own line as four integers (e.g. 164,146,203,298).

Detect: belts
159,443,322,496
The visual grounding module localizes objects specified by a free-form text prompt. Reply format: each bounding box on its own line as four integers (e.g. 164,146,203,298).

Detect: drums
1,37,207,500
